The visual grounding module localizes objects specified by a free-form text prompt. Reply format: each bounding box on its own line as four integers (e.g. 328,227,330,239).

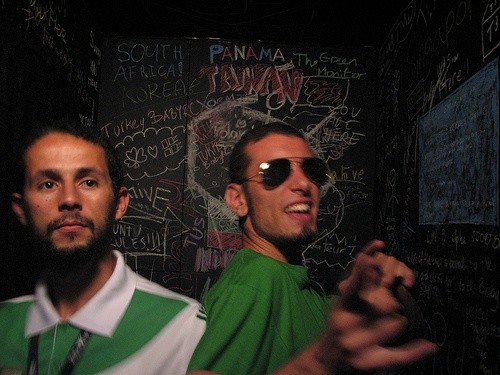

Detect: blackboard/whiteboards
0,0,500,375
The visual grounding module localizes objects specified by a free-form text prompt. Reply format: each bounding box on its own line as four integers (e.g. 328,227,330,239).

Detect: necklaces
27,327,92,375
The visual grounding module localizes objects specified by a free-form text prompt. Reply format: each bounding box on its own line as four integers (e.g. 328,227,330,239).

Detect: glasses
240,157,331,190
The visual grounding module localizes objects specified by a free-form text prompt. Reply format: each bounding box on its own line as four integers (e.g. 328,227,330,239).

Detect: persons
0,118,208,375
186,123,438,375
